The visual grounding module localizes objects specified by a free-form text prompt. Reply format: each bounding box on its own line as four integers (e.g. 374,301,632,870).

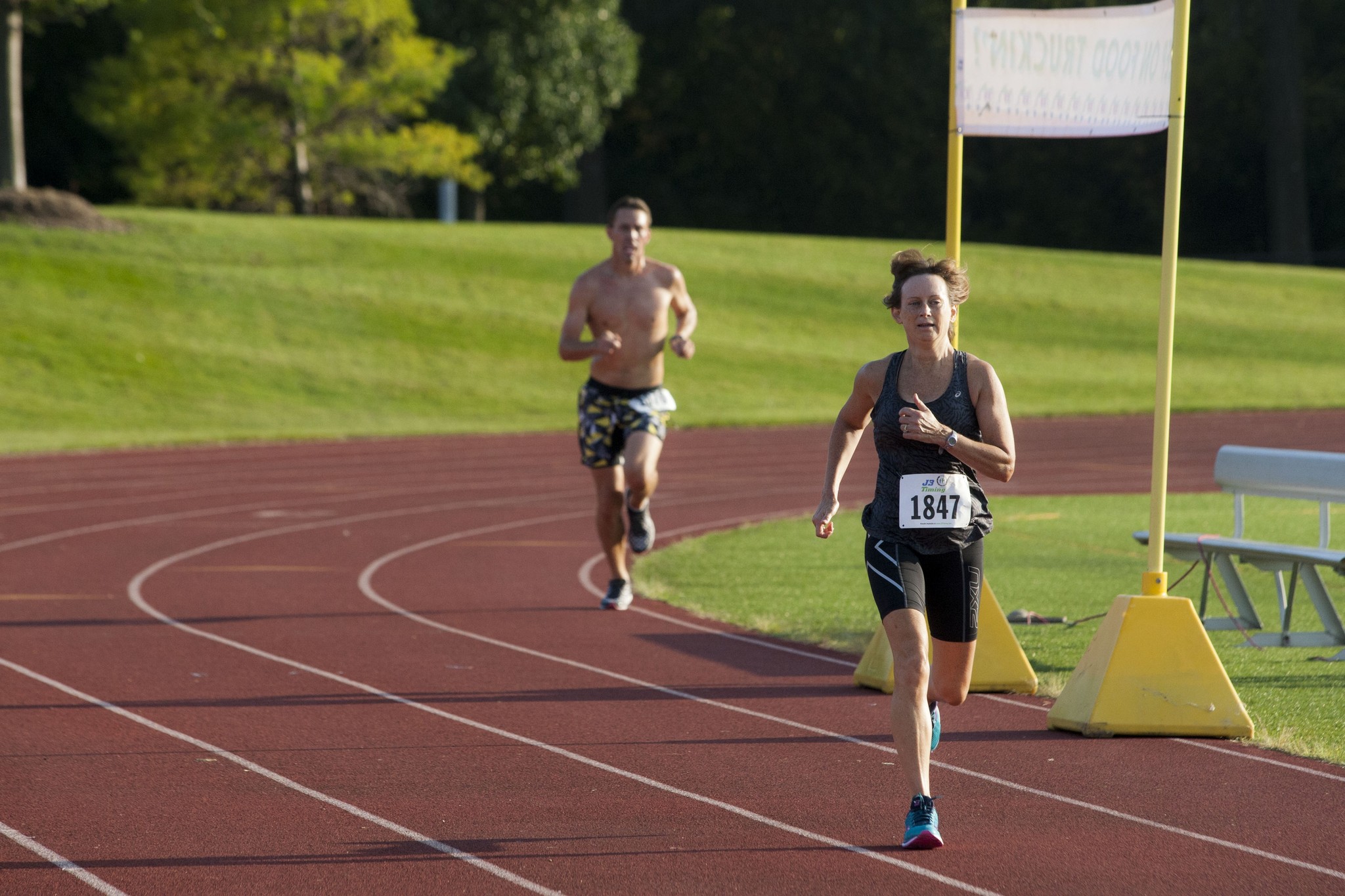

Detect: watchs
939,430,958,454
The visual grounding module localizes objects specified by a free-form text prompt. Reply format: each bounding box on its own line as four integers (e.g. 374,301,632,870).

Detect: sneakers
626,489,655,556
902,792,944,850
601,578,633,610
927,700,941,751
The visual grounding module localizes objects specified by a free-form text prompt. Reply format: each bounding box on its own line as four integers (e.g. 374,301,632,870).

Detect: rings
902,424,908,433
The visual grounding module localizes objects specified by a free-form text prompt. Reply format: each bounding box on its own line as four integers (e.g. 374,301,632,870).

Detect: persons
813,249,1015,848
559,197,697,610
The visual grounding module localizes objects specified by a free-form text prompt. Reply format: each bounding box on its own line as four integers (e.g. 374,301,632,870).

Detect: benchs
1131,445,1345,662
